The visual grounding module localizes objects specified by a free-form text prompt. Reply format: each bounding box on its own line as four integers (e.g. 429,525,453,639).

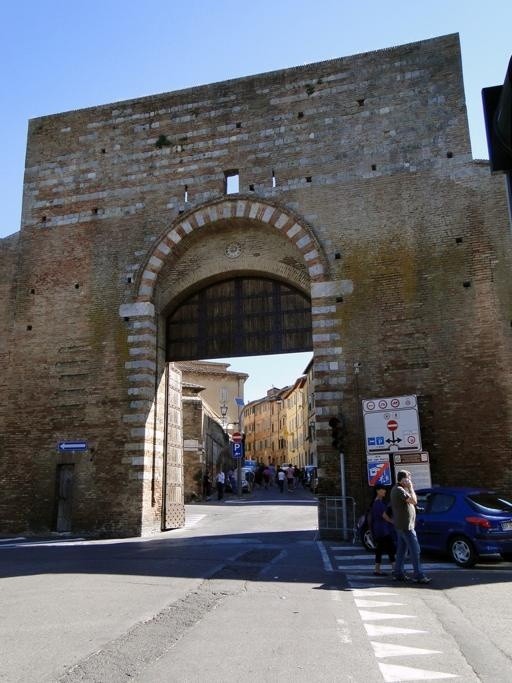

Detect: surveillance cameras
354,362,362,376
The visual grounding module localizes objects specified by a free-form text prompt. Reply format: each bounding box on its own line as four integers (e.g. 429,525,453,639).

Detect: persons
371,484,398,576
390,471,432,584
204,464,308,502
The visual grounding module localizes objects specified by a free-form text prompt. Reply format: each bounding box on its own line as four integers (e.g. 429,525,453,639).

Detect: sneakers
373,571,388,576
392,571,432,583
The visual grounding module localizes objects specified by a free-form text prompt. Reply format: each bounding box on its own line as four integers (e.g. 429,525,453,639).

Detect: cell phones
402,478,409,484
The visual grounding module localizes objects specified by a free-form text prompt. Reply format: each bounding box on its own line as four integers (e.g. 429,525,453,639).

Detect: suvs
353,487,512,568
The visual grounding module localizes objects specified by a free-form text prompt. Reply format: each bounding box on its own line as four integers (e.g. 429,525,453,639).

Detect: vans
301,465,318,486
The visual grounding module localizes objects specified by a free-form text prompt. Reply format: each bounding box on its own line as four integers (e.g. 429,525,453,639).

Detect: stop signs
232,432,242,443
386,419,398,432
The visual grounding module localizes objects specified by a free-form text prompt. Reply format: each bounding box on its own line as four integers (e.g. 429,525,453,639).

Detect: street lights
220,403,228,427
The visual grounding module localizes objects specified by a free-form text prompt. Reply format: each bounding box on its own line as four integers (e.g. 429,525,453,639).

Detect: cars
280,463,295,473
